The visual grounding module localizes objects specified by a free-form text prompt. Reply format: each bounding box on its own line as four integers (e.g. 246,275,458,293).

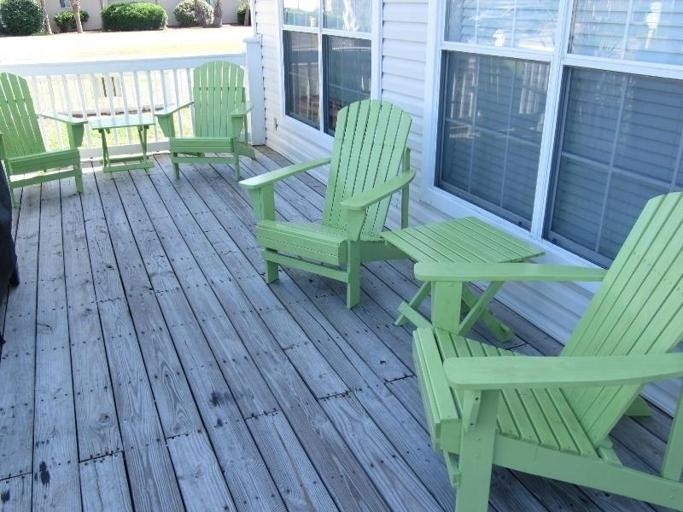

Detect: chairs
240,99,416,308
413,192,683,511
153,60,254,182
0,72,90,209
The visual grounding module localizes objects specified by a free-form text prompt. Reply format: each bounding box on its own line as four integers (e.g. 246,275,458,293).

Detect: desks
90,116,156,180
380,217,547,343
